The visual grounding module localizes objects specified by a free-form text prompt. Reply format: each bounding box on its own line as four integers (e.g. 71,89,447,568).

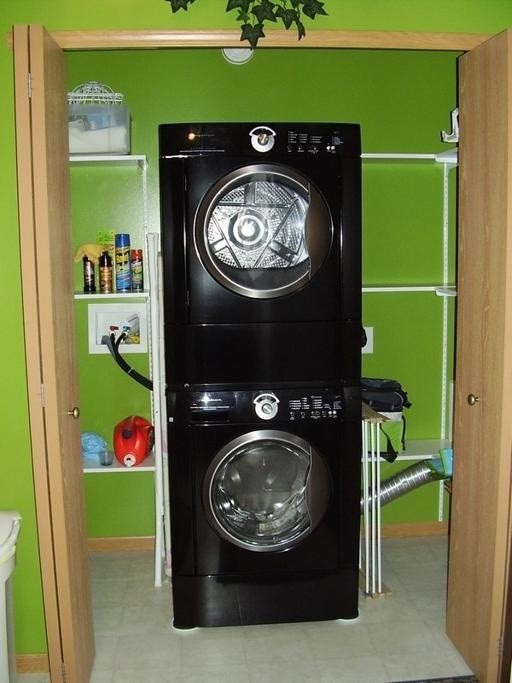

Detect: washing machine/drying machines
158,120,362,628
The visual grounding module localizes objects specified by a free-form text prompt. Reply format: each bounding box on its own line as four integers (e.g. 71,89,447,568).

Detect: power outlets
87,304,148,354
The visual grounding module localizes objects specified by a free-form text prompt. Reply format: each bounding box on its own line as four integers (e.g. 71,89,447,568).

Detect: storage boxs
63,79,131,155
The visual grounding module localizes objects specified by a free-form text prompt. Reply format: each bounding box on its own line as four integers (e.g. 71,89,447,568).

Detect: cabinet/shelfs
68,155,158,541
362,152,457,527
